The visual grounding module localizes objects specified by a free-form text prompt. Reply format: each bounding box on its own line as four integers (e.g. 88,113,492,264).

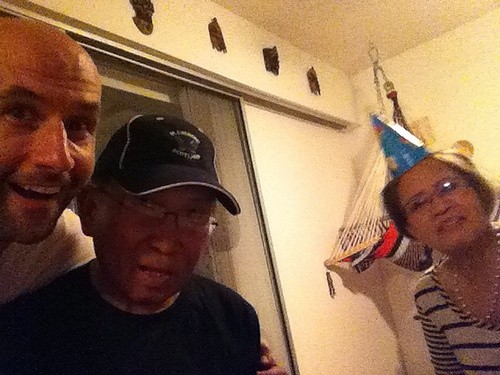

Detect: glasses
95,182,226,236
401,175,475,216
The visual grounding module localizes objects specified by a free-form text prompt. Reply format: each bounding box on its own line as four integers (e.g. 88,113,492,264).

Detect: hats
90,113,241,218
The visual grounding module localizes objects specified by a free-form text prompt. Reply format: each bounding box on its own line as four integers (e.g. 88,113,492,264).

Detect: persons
380,151,500,375
1,115,261,375
0,16,289,375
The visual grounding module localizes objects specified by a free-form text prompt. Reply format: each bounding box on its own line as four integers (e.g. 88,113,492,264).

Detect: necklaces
445,222,500,324
